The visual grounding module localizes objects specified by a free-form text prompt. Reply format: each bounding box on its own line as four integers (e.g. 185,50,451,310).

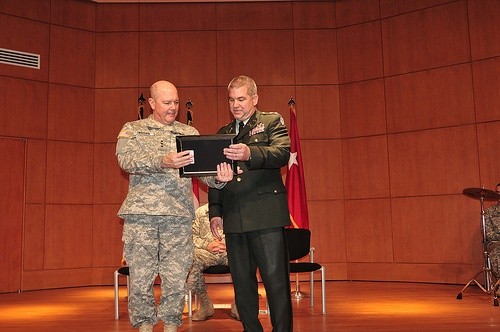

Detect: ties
238,121,244,133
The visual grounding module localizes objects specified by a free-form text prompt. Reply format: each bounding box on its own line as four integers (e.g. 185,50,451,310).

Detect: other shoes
163,323,178,332
138,323,153,332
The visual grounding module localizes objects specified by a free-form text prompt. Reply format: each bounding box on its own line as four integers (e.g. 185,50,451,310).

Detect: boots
192,290,214,322
231,296,241,320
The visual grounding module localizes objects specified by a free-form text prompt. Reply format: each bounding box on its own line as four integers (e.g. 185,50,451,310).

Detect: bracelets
249,155,252,164
215,180,222,185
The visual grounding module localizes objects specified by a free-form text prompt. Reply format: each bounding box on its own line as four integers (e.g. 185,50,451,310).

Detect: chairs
114,228,326,319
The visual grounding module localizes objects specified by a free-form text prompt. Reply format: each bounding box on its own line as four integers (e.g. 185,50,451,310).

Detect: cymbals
462,187,499,200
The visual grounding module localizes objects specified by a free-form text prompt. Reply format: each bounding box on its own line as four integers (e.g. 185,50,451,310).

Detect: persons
480,183,500,278
115,80,233,332
208,75,294,332
187,203,240,321
